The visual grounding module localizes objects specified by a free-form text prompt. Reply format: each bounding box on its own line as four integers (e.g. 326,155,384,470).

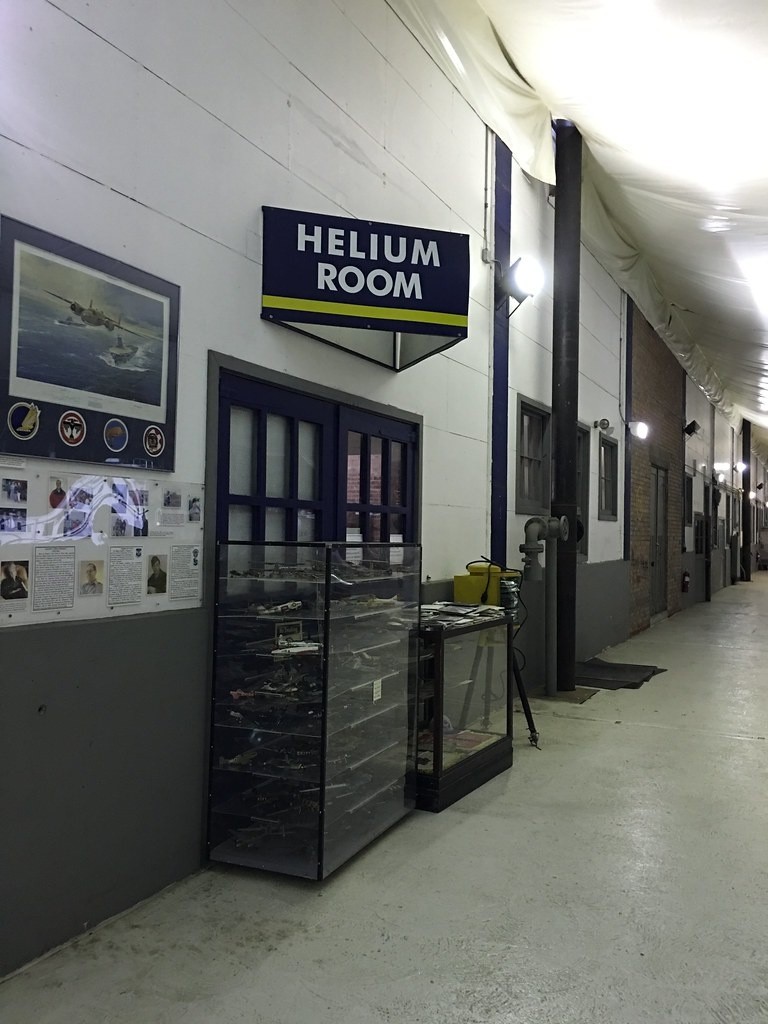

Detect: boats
109,334,139,364
42,288,147,338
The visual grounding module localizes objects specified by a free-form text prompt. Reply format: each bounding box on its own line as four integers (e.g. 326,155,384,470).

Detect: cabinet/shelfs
205,538,422,882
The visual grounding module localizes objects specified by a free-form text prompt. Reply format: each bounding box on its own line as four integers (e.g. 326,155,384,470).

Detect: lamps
629,422,649,439
683,420,701,442
757,483,763,490
714,473,724,484
496,256,544,318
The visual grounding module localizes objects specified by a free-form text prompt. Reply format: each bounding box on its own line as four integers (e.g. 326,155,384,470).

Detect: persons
148,556,167,594
1,561,28,599
6,480,23,502
135,515,148,536
79,563,102,594
50,479,67,508
0,510,26,530
